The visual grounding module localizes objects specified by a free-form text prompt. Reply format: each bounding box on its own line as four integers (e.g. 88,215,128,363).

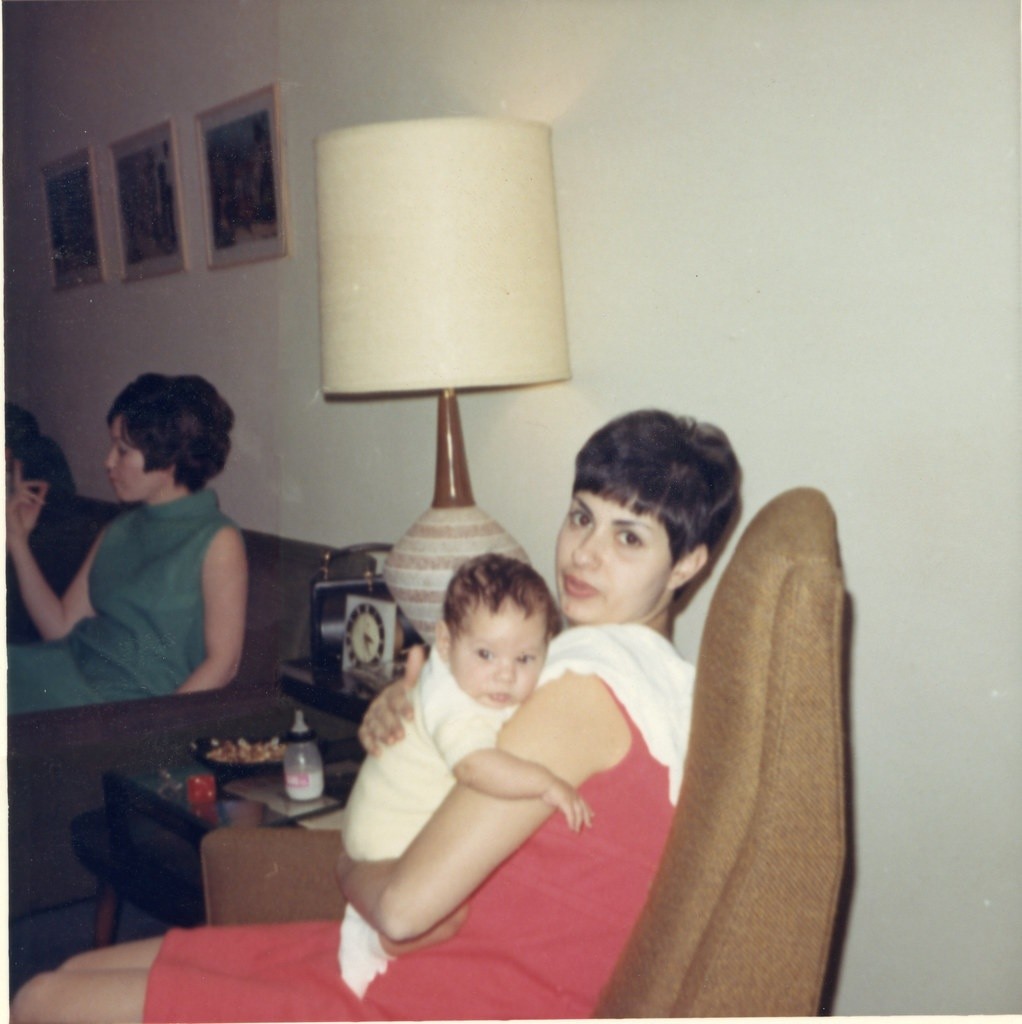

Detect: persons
5,371,745,1024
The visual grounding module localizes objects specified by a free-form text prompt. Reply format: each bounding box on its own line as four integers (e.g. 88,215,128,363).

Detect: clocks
342,592,396,693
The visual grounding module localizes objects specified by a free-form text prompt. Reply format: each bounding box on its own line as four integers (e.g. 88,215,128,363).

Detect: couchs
7,493,377,923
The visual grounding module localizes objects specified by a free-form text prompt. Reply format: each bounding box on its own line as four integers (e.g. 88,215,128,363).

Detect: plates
187,735,283,774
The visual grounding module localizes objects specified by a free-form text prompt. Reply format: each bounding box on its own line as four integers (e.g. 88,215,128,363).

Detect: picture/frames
194,82,289,269
40,146,106,293
107,113,189,284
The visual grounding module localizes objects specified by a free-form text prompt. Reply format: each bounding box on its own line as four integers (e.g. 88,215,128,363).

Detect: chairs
202,486,848,1018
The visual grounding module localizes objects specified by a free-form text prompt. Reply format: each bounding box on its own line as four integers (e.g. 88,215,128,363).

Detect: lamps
317,116,570,650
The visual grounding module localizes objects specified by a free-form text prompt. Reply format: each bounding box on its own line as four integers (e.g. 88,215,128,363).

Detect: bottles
282,709,325,799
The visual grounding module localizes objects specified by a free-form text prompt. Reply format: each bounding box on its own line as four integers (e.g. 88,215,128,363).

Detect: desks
75,659,376,952
